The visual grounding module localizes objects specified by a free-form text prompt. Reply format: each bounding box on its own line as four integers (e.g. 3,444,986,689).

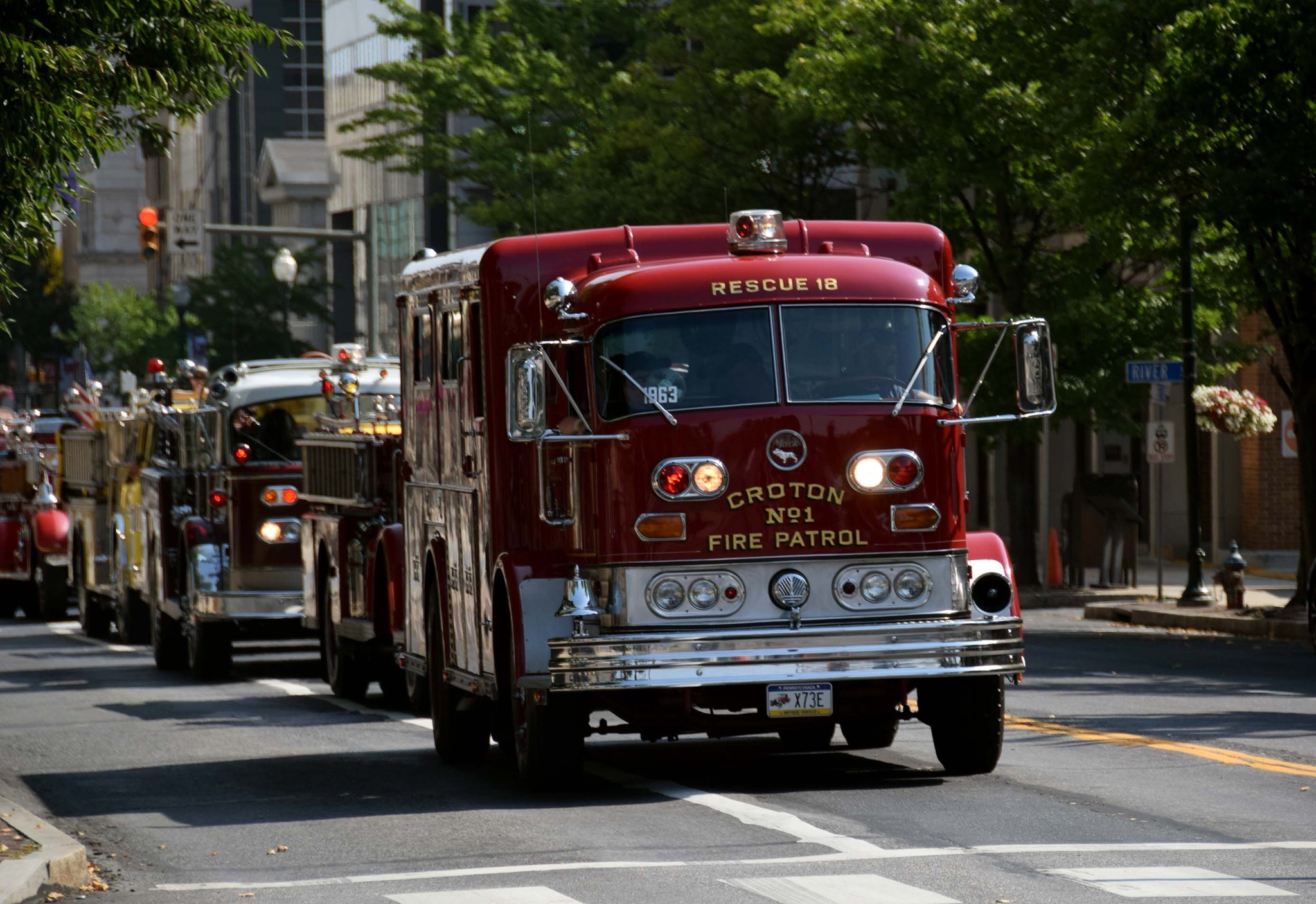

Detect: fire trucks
0,342,401,697
292,208,1057,785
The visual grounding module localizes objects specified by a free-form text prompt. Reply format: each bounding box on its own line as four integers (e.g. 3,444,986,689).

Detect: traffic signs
168,207,205,257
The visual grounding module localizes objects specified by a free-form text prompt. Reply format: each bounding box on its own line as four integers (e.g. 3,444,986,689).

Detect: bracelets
575,419,580,430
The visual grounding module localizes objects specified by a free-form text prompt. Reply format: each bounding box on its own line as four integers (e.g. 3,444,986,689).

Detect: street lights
272,246,297,359
173,279,193,360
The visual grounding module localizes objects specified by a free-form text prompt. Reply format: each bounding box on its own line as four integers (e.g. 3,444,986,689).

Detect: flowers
1191,384,1276,441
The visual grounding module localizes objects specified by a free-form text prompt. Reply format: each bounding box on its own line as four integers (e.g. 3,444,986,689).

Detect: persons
559,351,660,435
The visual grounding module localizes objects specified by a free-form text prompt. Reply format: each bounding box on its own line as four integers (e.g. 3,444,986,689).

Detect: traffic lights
138,205,168,259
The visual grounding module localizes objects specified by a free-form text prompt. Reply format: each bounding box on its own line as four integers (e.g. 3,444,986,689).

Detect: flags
74,388,93,428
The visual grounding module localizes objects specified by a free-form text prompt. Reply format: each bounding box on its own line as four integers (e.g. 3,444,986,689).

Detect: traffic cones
1045,530,1075,592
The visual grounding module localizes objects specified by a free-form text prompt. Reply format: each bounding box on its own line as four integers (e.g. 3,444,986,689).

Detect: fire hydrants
1216,539,1247,610
